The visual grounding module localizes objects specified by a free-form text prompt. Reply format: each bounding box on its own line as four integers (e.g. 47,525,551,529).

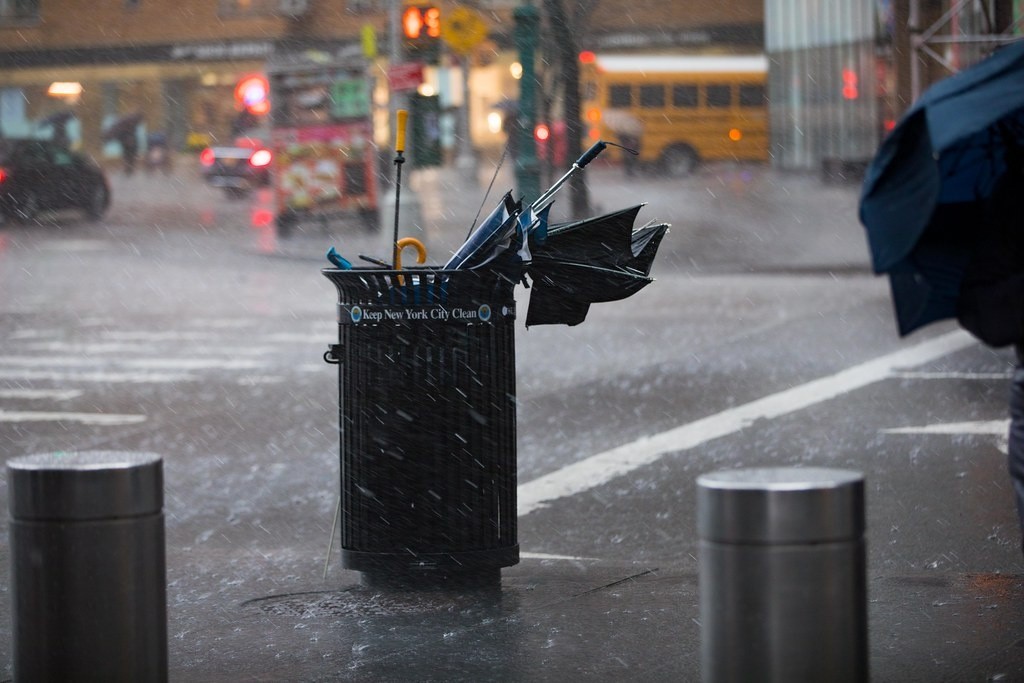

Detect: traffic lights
398,6,441,66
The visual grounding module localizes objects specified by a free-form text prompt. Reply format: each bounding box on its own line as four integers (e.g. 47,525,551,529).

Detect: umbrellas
605,111,643,135
327,112,668,327
40,105,80,127
491,100,520,110
101,114,143,139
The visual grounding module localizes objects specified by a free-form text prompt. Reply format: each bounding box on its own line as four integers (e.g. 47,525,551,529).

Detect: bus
552,54,776,177
272,63,389,236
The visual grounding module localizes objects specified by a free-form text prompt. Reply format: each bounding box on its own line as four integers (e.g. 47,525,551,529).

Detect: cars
203,125,278,198
0,138,110,221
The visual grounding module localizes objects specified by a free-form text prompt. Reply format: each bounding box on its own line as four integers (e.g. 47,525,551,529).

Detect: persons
54,121,69,148
120,125,137,174
619,132,640,177
504,111,520,159
